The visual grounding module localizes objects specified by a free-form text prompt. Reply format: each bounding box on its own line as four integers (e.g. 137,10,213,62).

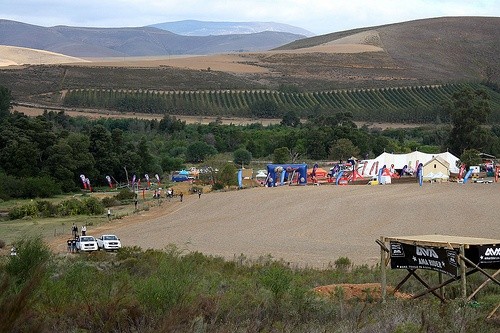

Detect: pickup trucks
96,234,122,252
75,236,98,252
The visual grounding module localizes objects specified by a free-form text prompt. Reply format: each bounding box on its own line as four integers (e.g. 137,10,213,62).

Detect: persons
67,223,86,254
180,192,183,202
107,208,111,218
152,188,175,199
134,194,138,209
198,190,201,199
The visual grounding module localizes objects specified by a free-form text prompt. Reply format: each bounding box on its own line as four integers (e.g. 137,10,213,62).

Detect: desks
379,232,500,302
